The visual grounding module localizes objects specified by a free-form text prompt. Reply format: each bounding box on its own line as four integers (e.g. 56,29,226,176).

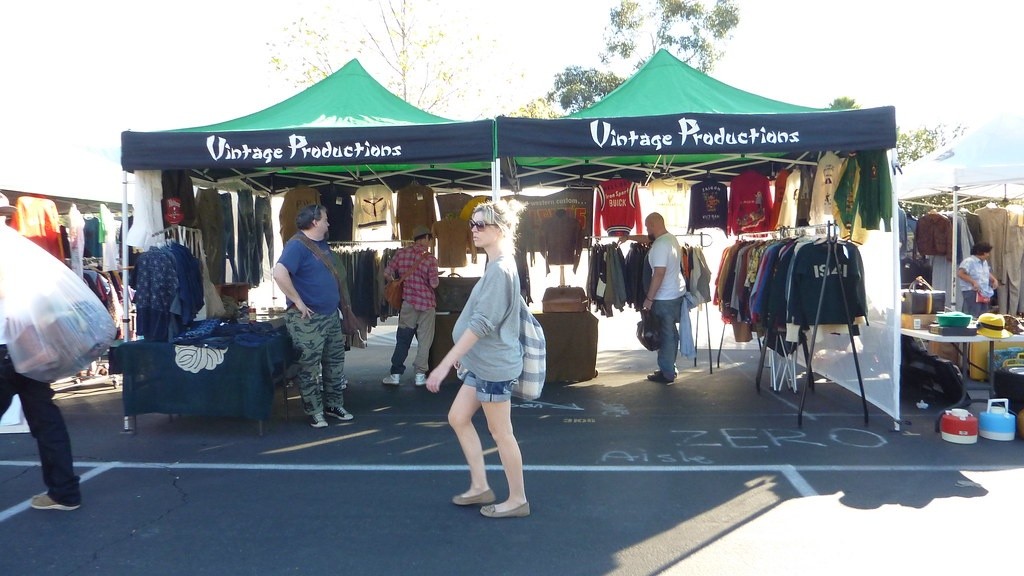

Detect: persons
641,212,687,383
382,225,440,385
426,200,531,518
0,222,84,510
271,203,355,426
957,241,999,315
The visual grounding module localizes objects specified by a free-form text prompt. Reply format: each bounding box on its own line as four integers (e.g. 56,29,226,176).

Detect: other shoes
452,490,496,505
481,501,530,518
647,370,675,383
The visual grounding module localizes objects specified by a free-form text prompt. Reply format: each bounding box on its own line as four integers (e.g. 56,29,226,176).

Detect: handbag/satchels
975,291,991,304
341,302,363,335
635,306,661,352
902,279,945,314
384,279,402,312
456,292,547,403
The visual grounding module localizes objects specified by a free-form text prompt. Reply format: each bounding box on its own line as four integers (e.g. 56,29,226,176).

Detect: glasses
468,218,497,232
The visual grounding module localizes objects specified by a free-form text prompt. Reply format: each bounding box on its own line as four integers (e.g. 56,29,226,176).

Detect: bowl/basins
936,314,972,328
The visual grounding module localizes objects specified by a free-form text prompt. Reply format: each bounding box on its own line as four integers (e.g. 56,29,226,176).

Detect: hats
974,312,1013,338
412,225,433,239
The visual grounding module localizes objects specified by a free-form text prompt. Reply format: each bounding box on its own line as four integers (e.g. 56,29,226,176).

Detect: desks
425,309,598,385
900,327,1024,433
114,319,300,438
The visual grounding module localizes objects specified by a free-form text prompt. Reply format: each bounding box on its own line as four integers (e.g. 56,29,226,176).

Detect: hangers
152,226,176,249
927,204,938,215
986,198,998,208
738,223,833,240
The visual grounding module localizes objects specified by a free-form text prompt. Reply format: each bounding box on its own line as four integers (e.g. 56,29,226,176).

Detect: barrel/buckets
941,409,978,444
978,399,1015,441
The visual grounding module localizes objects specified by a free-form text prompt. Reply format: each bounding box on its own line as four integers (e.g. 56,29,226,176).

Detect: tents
890,108,1024,317
494,47,902,432
123,58,491,435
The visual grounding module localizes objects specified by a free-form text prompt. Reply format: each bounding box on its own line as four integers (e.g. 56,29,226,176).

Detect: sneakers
382,373,400,386
309,411,328,427
31,492,80,511
414,375,427,386
325,405,353,420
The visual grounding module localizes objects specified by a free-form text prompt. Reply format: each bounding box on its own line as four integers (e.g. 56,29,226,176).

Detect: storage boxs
901,314,937,330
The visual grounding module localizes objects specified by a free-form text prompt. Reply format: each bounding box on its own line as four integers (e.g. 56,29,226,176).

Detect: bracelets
646,296,655,303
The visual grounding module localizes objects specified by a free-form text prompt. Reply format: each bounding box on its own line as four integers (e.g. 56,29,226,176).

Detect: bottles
272,296,278,314
249,302,256,322
1017,408,1024,439
